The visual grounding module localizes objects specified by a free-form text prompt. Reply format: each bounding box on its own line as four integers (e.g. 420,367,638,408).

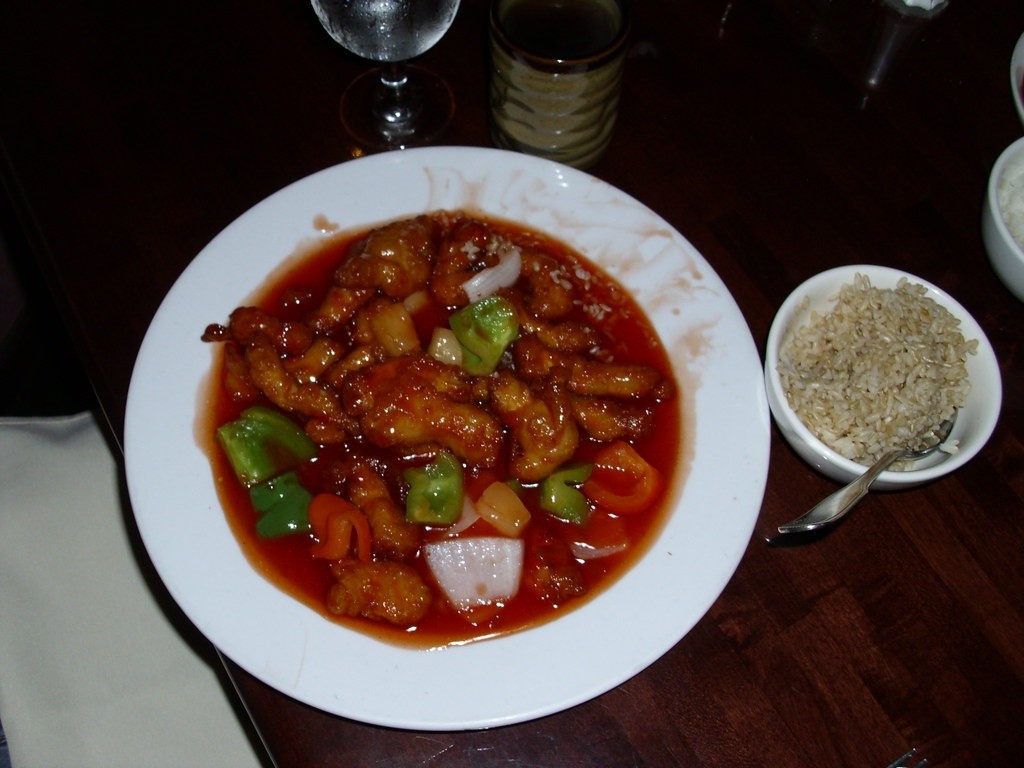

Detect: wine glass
311,0,461,149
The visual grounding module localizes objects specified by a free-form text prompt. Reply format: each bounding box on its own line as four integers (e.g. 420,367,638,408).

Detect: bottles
488,0,622,166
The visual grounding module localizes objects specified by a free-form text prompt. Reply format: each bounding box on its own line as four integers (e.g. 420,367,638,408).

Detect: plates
124,144,770,733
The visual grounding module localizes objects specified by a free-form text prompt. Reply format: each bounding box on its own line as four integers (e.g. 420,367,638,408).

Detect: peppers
215,294,663,561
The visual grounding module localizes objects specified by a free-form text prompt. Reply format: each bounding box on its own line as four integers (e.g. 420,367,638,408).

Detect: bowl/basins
764,263,1002,492
985,135,1024,301
1009,31,1024,123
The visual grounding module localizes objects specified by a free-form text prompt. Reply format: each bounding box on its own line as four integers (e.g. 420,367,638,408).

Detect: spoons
777,405,958,535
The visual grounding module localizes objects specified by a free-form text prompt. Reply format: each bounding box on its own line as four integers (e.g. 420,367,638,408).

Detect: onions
461,247,522,302
422,537,524,610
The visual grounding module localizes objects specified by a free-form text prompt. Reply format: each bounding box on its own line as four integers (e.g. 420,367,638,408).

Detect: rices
777,272,979,472
